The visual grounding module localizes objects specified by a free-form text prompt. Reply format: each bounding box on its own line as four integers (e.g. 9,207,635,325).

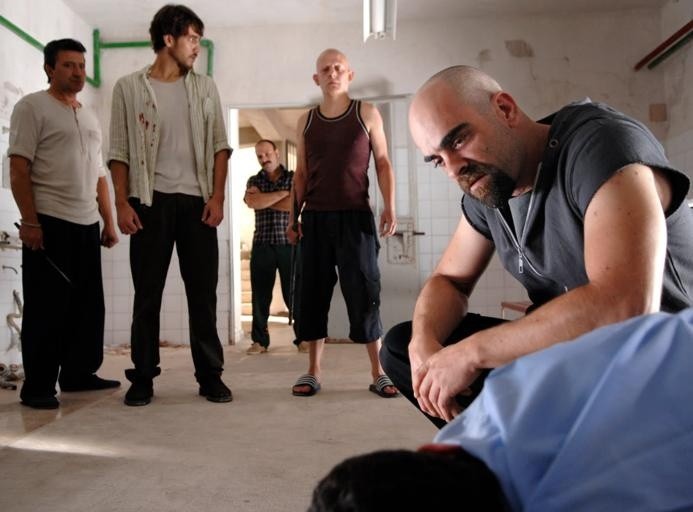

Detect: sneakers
56,373,121,393
197,377,235,403
296,340,311,354
123,377,156,408
245,341,269,355
18,386,63,410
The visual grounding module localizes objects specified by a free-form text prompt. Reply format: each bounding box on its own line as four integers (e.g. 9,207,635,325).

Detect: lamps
361,0,398,48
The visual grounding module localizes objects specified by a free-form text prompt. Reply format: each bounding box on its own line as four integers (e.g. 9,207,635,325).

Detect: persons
7,38,121,410
306,303,693,512
284,48,404,398
378,66,693,431
105,5,233,407
242,140,310,356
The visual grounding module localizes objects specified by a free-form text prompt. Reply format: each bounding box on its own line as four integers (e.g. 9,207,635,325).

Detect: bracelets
19,218,42,229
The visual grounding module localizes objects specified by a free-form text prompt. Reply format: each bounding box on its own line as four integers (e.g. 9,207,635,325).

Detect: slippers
289,374,325,398
365,375,401,399
